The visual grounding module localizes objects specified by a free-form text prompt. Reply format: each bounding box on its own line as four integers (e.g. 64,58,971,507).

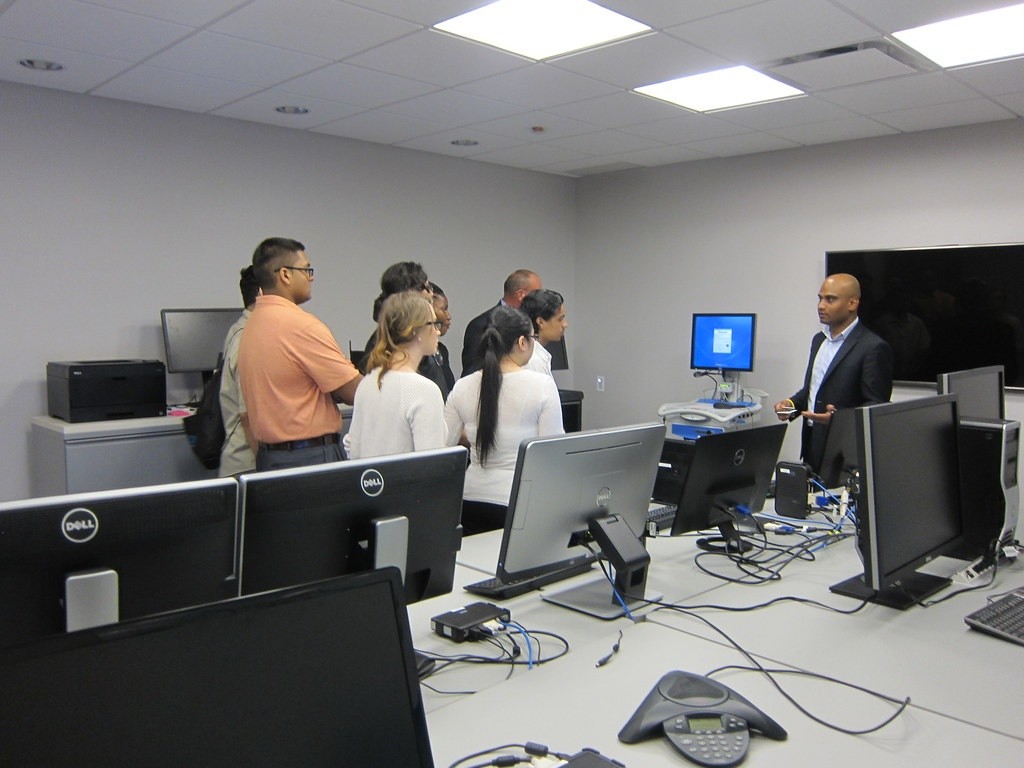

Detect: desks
407,492,1024,768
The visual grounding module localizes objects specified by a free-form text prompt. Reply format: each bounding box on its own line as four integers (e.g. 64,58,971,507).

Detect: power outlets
596,376,606,392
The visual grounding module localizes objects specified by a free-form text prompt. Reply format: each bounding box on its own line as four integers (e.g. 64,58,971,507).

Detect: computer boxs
940,416,1020,560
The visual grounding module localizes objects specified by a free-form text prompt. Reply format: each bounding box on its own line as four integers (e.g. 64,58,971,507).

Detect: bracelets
787,399,795,408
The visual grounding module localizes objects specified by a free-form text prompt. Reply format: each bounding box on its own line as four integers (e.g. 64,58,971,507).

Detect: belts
258,431,340,451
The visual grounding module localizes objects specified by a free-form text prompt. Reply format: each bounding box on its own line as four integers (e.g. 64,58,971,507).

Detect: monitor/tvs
670,421,789,554
544,334,568,369
161,307,244,391
494,421,668,618
690,313,757,370
828,392,960,611
937,366,1005,421
827,242,1024,391
0,447,470,768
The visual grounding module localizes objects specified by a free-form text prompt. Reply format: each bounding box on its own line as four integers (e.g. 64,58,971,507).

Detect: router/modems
774,461,813,519
430,602,511,644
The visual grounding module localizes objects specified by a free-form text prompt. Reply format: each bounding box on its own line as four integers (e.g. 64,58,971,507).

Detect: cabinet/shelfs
31,403,355,499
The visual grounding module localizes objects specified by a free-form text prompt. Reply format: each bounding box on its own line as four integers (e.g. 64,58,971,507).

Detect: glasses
274,265,314,277
427,321,443,330
515,334,539,344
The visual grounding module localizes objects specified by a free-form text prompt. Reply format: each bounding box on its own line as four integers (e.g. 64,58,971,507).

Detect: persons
444,305,565,537
361,261,448,405
343,291,449,459
218,265,260,477
521,290,567,378
238,238,364,473
461,270,542,377
427,281,456,391
774,273,893,486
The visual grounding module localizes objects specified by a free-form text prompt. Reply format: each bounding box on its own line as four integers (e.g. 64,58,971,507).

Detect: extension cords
963,554,994,582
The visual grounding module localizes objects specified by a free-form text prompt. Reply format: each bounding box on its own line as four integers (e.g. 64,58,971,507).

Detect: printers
46,360,167,423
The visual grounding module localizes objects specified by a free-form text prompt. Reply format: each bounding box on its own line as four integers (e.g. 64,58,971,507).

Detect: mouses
713,403,730,409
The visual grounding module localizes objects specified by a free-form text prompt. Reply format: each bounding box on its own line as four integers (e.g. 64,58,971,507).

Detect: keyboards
965,586,1024,647
645,504,680,531
463,556,600,600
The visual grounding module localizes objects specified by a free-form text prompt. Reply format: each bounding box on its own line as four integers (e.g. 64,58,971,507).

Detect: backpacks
182,327,244,471
